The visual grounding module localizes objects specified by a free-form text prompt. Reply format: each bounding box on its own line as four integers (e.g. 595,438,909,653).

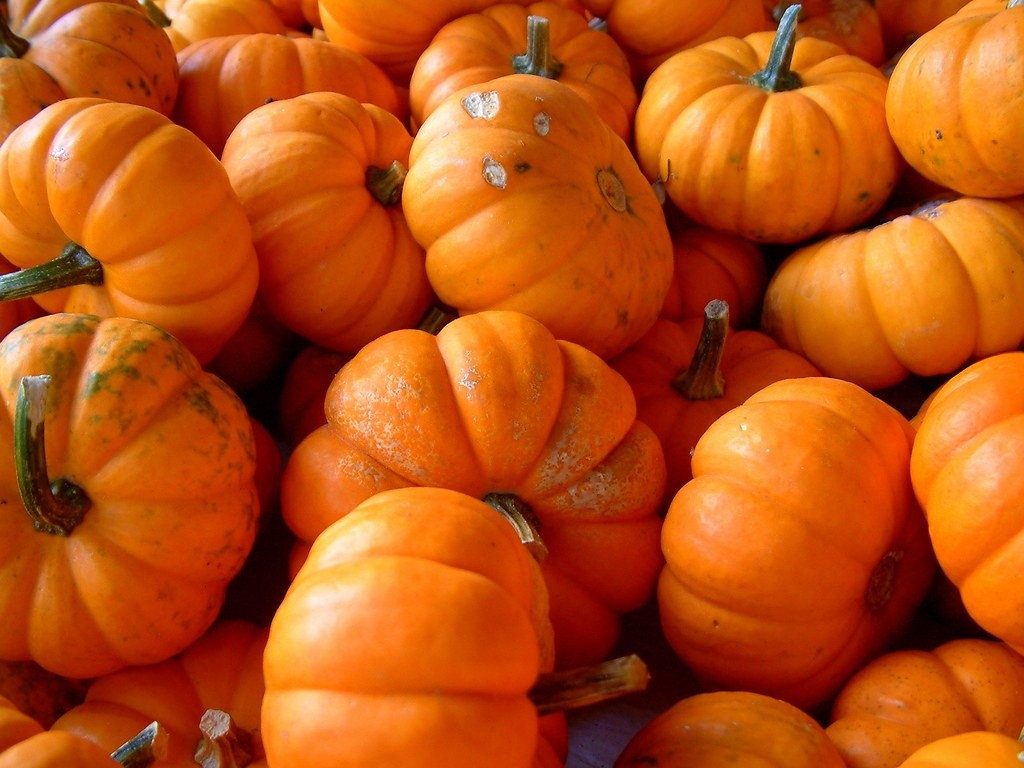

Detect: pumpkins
0,0,1023,768
